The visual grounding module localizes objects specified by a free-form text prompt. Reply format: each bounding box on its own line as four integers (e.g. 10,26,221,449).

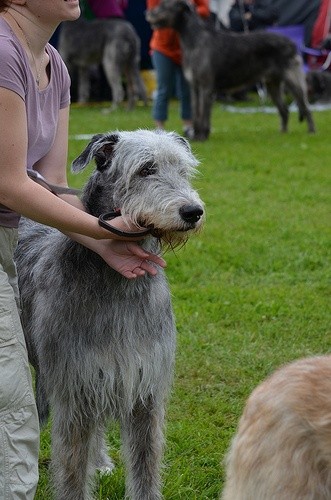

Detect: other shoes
184,127,196,139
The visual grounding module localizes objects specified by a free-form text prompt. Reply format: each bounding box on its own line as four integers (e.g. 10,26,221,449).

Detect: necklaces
4,9,46,86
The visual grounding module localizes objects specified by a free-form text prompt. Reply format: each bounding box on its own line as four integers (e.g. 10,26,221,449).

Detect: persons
145,0,209,138
1,0,166,499
228,0,257,31
92,0,126,19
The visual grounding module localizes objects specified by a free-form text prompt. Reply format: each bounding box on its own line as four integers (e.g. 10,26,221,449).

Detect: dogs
144,0,331,141
58,1,149,115
216,353,331,500
12,126,207,500
